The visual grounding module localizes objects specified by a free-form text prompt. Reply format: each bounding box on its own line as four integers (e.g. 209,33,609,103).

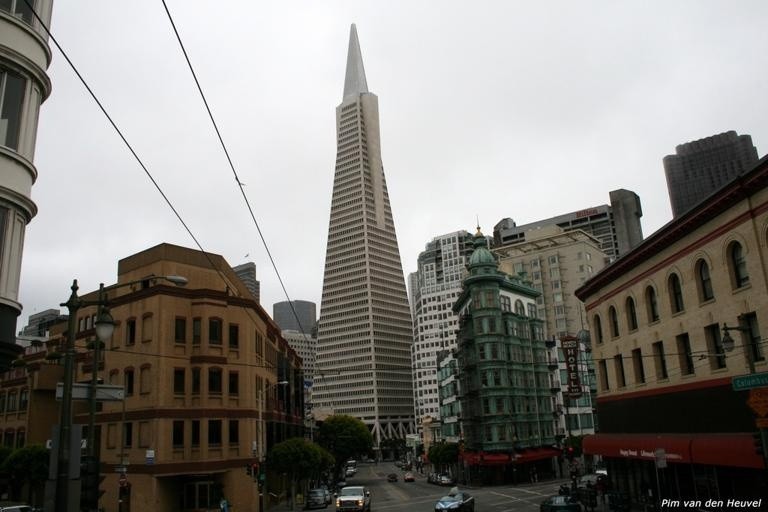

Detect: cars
572,473,611,496
387,472,416,482
540,495,581,512
425,472,456,485
435,490,474,512
334,483,369,509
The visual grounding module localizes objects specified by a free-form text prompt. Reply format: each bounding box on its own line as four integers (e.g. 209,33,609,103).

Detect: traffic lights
567,446,574,461
246,462,258,479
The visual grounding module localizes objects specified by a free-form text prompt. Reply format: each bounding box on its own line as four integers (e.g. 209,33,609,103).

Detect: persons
557,477,594,496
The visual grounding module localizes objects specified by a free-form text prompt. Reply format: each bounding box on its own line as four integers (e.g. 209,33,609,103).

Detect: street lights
55,276,188,512
258,379,289,511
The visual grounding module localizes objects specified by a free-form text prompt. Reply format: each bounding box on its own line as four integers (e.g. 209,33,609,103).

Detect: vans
307,488,332,509
